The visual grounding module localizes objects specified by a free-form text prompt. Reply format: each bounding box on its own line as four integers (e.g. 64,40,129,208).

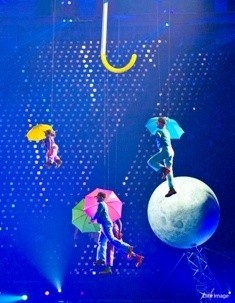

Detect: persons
147,118,177,197
45,131,63,169
97,218,144,275
91,192,135,266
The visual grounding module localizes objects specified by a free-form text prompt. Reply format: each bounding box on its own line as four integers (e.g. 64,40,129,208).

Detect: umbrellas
71,199,100,233
83,188,122,222
26,124,52,141
144,116,184,139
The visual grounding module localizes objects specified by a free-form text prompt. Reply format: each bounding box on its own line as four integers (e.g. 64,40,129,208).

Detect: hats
94,192,106,198
158,118,165,124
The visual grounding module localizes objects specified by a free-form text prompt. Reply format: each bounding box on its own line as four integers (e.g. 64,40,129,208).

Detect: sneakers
162,168,170,179
128,246,134,259
99,271,112,275
93,261,105,265
136,255,144,268
165,189,176,197
